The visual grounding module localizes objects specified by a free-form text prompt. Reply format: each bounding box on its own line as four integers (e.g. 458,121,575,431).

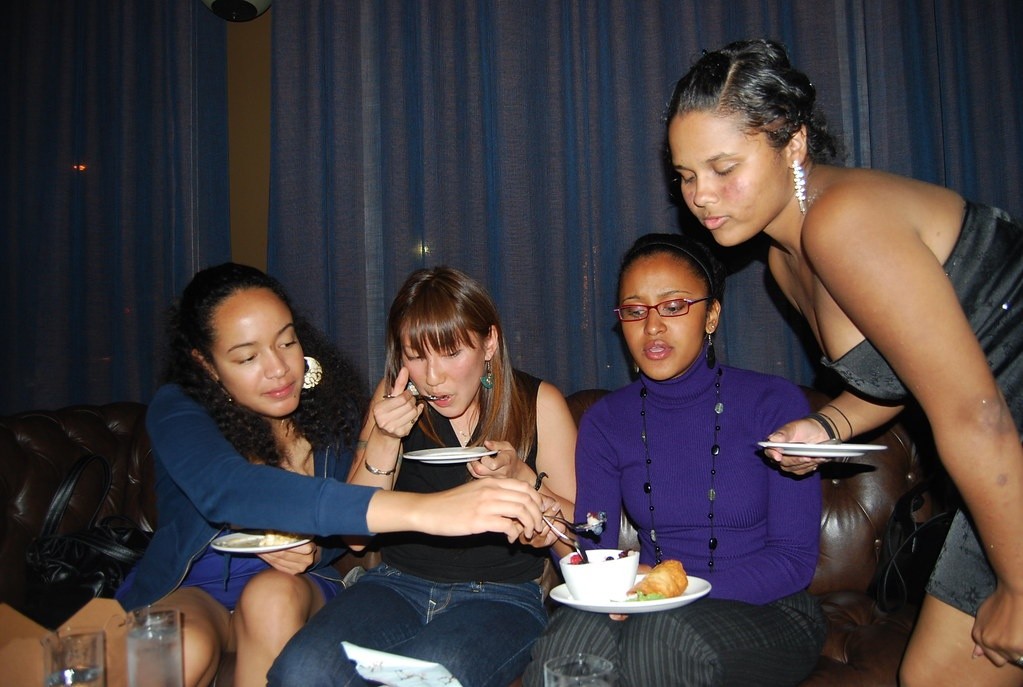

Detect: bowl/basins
559,549,640,600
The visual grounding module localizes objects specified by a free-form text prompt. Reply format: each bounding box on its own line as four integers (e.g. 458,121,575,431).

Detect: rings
1016,657,1023,667
410,420,416,425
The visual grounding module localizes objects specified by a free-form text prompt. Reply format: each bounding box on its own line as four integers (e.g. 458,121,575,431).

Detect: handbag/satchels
876,480,957,613
26,454,159,631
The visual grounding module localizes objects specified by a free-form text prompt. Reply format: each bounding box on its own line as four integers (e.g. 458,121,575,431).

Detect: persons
111,263,547,686
508,233,821,687
263,268,580,687
664,38,1023,687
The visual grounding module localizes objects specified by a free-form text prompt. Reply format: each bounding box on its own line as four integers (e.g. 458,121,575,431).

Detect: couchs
0,387,932,687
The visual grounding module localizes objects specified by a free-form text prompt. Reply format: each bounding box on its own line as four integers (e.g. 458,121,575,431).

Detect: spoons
542,513,606,534
541,515,588,564
383,395,447,402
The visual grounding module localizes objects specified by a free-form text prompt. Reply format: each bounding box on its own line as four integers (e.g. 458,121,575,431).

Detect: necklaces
452,420,476,448
274,419,294,442
641,366,722,575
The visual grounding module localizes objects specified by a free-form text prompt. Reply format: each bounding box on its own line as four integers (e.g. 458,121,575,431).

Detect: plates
549,574,711,613
210,531,315,553
758,441,888,457
402,447,498,463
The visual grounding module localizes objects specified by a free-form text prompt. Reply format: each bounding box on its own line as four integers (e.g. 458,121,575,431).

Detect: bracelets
534,471,548,495
807,403,854,445
364,459,396,479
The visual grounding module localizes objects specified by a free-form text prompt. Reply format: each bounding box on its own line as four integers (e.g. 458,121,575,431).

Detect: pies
623,560,689,602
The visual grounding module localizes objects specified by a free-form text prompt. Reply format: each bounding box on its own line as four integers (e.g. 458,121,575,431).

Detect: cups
126,606,185,687
543,653,613,687
44,625,106,687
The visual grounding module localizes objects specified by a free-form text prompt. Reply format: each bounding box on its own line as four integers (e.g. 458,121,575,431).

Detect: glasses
613,297,711,322
